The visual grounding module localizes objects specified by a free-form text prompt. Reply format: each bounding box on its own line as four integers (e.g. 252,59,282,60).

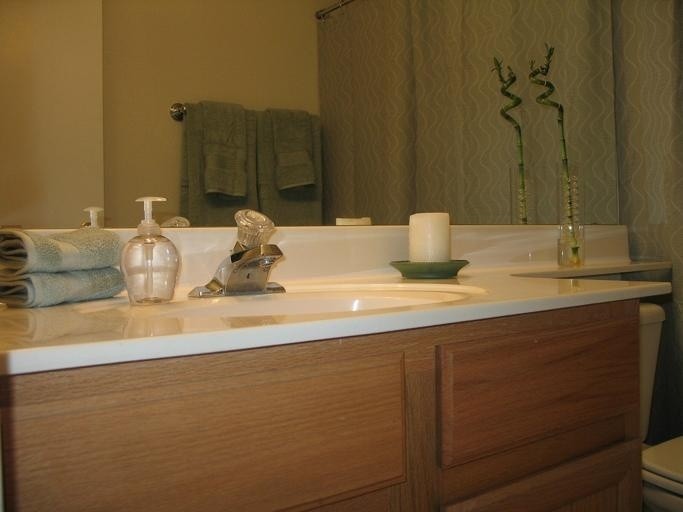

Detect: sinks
155,281,486,318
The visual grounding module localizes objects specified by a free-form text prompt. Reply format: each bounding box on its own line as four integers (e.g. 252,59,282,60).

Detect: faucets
187,208,287,298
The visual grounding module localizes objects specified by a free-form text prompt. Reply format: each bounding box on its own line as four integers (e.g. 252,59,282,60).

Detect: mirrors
0,0,623,229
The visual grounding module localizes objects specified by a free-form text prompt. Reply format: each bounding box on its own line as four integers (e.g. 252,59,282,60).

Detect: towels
0,225,127,308
177,99,332,226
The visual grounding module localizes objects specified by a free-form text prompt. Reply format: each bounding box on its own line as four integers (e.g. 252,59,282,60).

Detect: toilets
638,302,683,512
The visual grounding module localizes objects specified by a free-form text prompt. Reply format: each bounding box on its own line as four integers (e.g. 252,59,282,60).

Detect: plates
390,261,468,279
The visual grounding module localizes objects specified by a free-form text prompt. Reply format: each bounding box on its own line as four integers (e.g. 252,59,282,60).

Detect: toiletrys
83,206,105,227
119,196,181,303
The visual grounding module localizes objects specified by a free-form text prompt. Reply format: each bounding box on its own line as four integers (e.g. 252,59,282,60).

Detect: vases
554,166,584,268
509,160,536,224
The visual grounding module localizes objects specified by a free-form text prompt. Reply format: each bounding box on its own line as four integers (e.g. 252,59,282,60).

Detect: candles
334,217,372,225
407,212,451,262
